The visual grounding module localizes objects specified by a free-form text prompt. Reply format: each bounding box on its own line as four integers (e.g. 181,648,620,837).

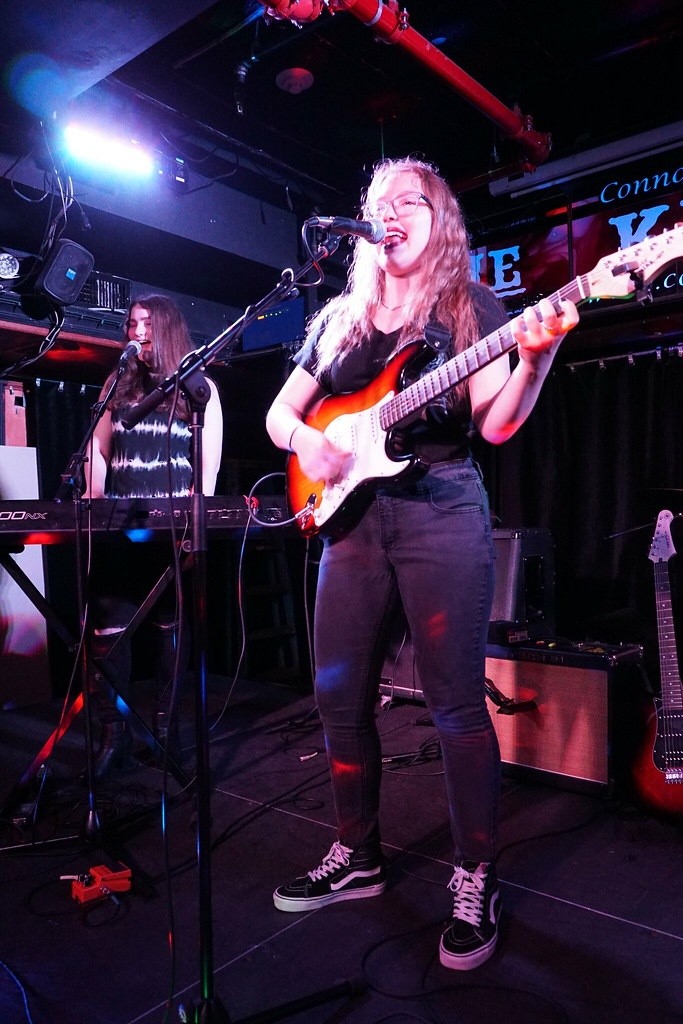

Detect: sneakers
439,859,503,970
273,835,388,912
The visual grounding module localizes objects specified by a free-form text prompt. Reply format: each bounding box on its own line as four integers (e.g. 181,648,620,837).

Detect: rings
543,321,558,330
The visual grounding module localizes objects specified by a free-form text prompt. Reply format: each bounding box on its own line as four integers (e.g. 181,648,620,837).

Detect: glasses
369,192,434,216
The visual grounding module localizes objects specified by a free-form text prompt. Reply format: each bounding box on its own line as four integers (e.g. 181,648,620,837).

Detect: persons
73,292,223,784
265,155,581,971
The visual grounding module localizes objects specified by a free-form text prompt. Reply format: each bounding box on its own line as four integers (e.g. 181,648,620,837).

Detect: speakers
480,637,644,788
374,526,553,699
31,238,95,309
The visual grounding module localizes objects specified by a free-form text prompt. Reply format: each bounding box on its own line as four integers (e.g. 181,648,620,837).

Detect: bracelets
288,422,304,453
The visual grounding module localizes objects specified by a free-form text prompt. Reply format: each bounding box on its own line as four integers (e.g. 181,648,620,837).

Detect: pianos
0,496,297,543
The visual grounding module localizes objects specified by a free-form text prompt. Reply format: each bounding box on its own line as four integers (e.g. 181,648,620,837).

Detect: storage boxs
484,657,650,802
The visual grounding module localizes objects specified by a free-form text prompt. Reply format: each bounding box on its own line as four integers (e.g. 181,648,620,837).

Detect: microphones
121,340,143,360
498,697,540,715
305,216,387,245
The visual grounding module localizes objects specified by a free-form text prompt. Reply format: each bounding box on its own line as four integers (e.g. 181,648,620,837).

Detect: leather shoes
153,713,181,772
93,720,132,783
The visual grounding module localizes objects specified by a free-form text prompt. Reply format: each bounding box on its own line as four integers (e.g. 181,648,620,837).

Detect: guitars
285,221,683,541
626,508,683,826
